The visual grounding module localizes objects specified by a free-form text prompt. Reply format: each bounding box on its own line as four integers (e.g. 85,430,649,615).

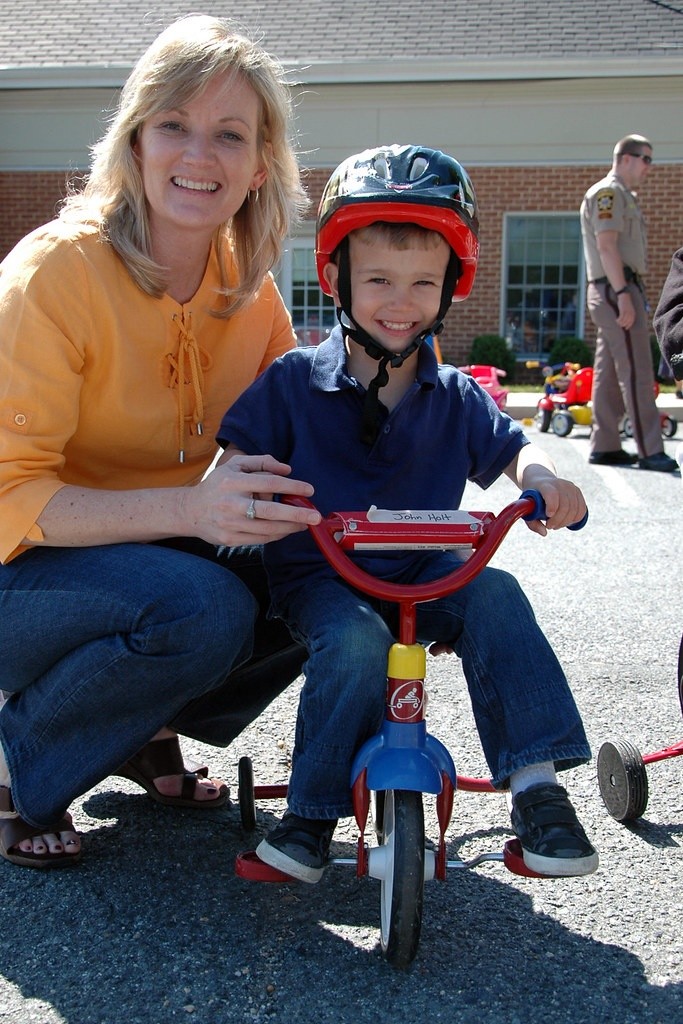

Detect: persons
0,14,322,867
655,247,683,710
579,134,678,473
215,146,598,885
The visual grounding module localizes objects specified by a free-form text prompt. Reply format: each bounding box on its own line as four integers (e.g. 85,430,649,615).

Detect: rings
248,499,255,519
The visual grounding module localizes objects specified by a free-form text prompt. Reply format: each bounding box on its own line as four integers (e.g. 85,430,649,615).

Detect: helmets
315,144,479,302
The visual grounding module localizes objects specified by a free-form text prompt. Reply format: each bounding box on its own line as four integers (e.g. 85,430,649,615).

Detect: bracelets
616,286,630,295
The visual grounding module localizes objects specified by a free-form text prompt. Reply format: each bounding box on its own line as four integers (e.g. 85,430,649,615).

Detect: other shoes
639,452,679,471
589,450,639,465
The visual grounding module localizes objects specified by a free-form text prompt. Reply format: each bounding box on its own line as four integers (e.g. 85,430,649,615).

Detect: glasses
617,152,652,164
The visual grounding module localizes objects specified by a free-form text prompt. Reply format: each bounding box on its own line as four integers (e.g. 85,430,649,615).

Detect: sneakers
256,808,338,884
511,785,599,875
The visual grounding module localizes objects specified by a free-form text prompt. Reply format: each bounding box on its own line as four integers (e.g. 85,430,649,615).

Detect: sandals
0,786,81,867
110,735,229,807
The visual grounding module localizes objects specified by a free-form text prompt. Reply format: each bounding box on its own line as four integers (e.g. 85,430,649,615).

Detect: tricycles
597,635,683,823
237,487,589,962
525,361,594,438
618,384,677,439
459,366,508,411
